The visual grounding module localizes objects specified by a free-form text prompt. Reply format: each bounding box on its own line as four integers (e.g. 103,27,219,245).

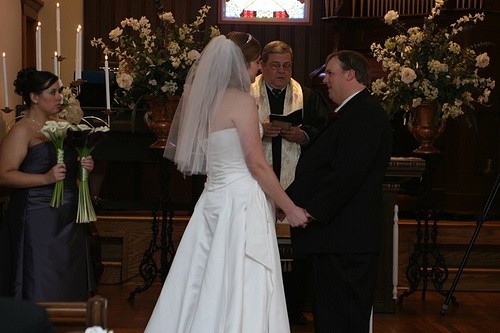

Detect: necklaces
33,120,42,123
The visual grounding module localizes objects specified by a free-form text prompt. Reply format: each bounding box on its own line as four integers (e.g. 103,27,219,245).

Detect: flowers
369,0,496,120
91,5,220,110
42,121,79,208
73,125,110,223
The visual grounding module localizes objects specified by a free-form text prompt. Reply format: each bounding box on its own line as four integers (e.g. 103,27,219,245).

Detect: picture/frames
218,0,312,26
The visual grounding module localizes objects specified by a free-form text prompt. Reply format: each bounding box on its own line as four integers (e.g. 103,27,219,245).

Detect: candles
79,30,82,79
38,24,41,71
36,30,38,70
2,55,9,107
54,55,58,76
58,61,60,79
105,60,110,109
75,31,80,80
56,6,61,56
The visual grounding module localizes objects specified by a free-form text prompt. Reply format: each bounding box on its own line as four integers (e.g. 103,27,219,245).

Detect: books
269,109,302,131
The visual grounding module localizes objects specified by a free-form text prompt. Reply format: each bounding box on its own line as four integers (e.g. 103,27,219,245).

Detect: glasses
265,63,292,69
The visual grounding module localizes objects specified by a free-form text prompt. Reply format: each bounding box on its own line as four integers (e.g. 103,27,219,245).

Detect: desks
397,154,458,305
128,149,176,302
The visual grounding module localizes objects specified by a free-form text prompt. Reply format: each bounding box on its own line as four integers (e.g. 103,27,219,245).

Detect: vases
408,101,441,154
146,95,181,148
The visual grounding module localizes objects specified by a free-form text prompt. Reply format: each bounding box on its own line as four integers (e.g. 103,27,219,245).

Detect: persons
0,67,104,303
275,51,393,333
145,32,307,333
249,41,328,307
